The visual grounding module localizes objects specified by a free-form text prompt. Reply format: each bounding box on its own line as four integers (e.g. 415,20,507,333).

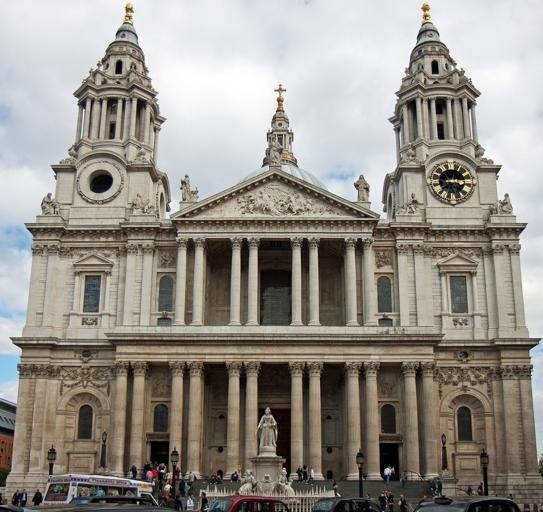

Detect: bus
40,473,158,506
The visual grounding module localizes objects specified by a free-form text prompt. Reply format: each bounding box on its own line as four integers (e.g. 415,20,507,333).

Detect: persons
268,137,284,166
0,493,3,505
129,461,239,512
296,464,543,512
237,407,296,495
41,193,52,216
12,489,21,507
20,489,27,507
499,193,513,214
132,192,150,212
180,173,191,202
408,193,418,214
354,175,370,202
32,489,43,506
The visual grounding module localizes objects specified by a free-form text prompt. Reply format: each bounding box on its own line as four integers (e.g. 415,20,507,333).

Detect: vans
412,495,520,511
70,495,155,506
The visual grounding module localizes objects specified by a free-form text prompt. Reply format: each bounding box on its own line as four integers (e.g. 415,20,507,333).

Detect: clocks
428,160,478,207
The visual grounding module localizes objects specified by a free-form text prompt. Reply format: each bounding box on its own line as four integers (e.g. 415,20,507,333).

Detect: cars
206,494,290,511
0,502,174,512
311,496,384,511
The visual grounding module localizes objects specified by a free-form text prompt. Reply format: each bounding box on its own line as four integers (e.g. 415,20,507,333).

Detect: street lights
101,431,107,468
169,445,179,496
479,447,489,496
46,444,56,474
354,447,364,497
439,432,448,475
